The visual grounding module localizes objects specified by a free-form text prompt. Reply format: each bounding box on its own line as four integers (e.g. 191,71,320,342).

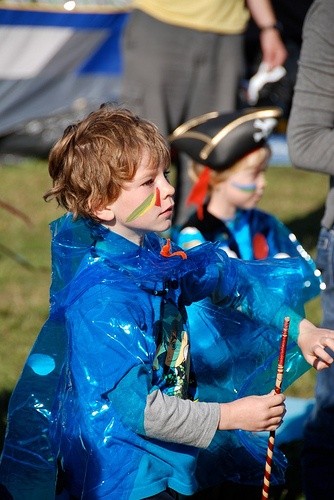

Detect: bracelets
259,23,280,32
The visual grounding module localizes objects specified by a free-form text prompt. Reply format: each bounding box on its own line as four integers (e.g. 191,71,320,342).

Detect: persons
0,108,334,500
123,0,286,226
287,1,334,440
168,110,325,485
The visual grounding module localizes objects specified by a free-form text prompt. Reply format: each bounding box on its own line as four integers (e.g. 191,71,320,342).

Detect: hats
167,106,283,172
